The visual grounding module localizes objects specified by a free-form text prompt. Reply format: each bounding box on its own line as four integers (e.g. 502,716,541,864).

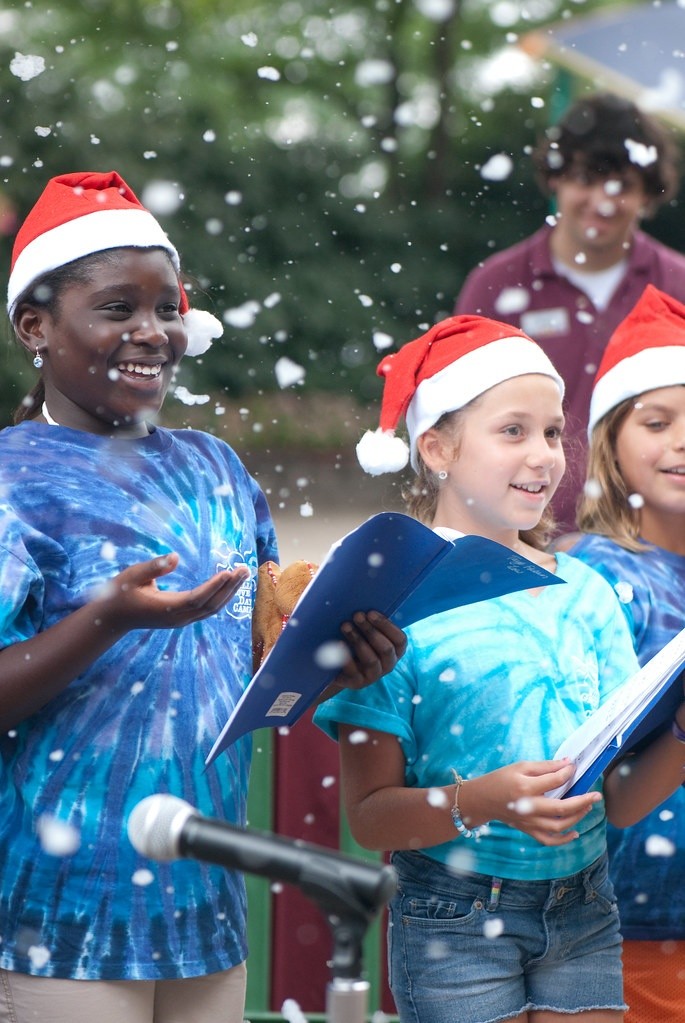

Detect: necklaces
42,401,58,426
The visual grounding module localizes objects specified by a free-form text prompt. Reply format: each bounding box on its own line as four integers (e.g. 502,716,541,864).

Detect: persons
453,97,684,553
313,315,685,1022
0,170,409,1023
571,285,685,1022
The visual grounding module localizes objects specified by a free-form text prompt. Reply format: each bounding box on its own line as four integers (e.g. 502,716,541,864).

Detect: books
202,512,568,777
548,628,685,800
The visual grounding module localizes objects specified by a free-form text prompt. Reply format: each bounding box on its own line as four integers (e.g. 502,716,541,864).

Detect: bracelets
670,715,685,743
451,768,489,839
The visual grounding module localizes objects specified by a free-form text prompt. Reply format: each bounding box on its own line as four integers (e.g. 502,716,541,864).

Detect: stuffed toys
251,559,318,668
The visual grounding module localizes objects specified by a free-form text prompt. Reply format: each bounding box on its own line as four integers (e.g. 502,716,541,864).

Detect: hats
9,172,223,358
357,313,565,476
587,282,685,446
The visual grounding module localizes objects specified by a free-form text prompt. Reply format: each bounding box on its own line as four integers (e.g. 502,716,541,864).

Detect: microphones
127,793,400,910
